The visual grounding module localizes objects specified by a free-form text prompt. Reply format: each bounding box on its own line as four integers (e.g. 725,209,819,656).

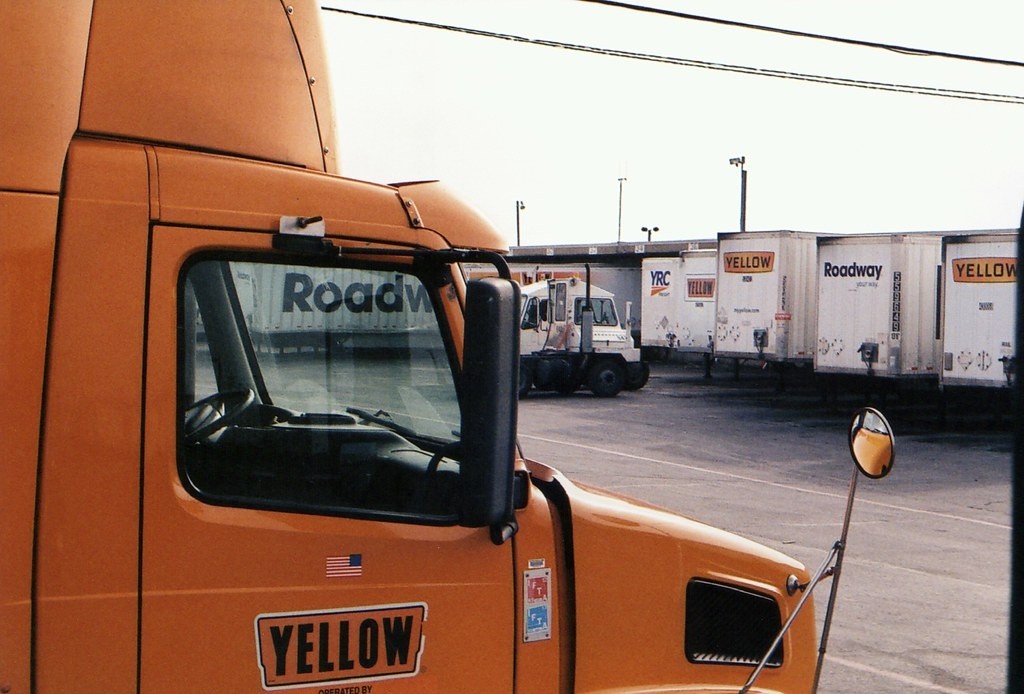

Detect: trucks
0,1,897,694
517,264,649,398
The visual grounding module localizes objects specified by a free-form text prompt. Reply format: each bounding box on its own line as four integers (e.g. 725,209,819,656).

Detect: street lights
516,201,525,246
640,226,659,242
728,155,747,232
618,176,627,242
